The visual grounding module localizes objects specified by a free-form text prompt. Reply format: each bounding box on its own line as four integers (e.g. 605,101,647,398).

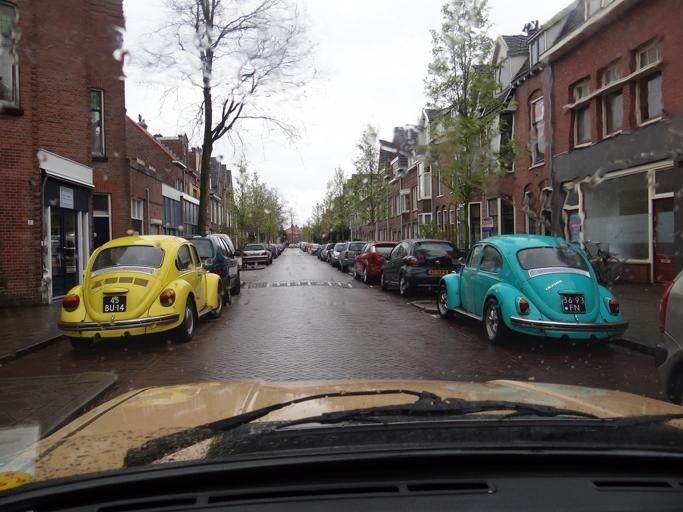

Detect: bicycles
569,237,626,284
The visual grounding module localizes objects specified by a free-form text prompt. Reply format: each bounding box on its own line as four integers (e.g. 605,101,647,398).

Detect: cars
286,236,460,300
57,231,230,353
183,234,288,301
652,262,682,408
436,232,631,348
